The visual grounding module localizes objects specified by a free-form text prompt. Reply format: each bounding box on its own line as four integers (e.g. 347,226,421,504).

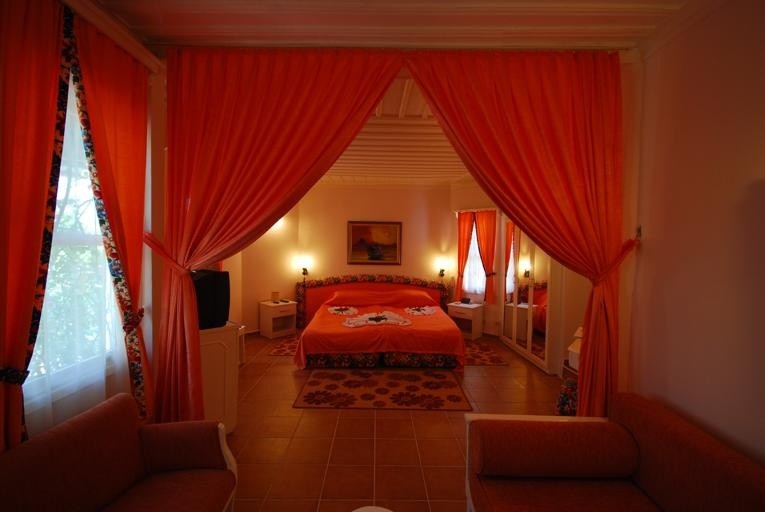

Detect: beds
519,282,547,334
294,274,466,369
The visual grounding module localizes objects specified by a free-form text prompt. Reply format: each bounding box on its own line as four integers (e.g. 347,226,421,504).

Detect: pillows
324,289,440,308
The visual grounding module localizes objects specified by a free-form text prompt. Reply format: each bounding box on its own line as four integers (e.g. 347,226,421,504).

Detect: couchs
465,391,765,512
0,393,239,512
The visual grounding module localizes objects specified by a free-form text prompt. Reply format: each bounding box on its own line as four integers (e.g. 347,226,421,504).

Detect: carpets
292,369,473,411
268,336,298,356
464,339,509,366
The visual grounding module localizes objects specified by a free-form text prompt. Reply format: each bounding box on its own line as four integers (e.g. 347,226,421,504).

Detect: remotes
280,299,289,303
273,301,279,304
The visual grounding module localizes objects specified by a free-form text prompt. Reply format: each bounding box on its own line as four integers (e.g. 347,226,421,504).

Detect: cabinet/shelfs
199,321,241,435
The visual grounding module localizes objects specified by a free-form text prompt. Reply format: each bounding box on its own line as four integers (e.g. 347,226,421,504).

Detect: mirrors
503,217,551,362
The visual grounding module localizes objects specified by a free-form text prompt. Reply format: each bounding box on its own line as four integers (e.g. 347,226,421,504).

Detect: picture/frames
347,220,401,266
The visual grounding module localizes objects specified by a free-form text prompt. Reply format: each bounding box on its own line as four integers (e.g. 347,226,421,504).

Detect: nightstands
447,301,484,341
504,302,538,341
259,299,298,340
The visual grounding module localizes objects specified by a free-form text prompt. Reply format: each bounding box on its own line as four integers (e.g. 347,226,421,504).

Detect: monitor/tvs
190,269,230,330
